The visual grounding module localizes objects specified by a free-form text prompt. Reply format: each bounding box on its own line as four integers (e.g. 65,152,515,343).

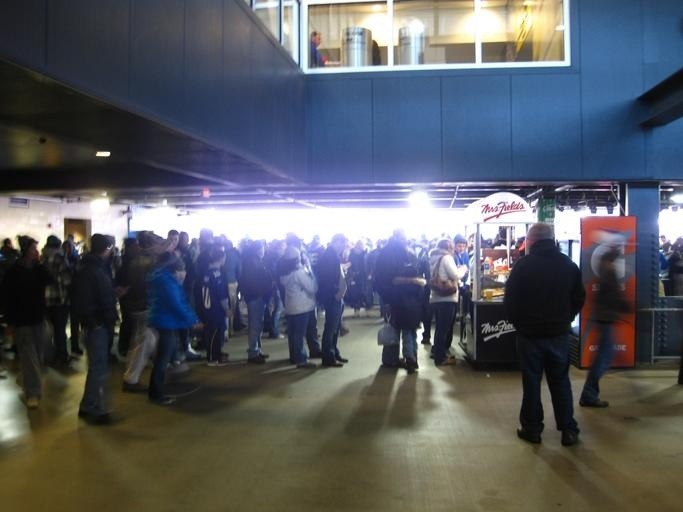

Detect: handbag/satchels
462,286,472,299
428,255,457,297
377,312,401,347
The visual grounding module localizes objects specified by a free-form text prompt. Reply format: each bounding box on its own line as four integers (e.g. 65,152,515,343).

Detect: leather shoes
517,425,542,445
561,428,580,446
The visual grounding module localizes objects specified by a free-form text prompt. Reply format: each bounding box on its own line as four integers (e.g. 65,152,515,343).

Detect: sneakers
248,354,269,365
205,358,230,367
310,350,325,358
110,353,121,360
297,362,317,369
78,408,96,419
71,345,84,356
579,397,611,409
148,391,178,405
340,327,350,336
321,357,349,367
435,357,457,365
269,332,285,339
93,412,126,428
24,392,42,408
406,365,417,374
185,352,202,360
380,361,406,368
122,380,150,392
421,338,431,344
16,375,28,390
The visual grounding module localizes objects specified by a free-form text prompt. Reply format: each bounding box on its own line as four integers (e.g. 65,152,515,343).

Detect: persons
468,233,485,285
67,234,80,257
190,228,215,360
417,239,428,261
430,235,472,359
115,238,140,355
189,238,200,262
490,230,507,248
197,253,235,367
308,236,325,312
147,252,208,405
120,234,169,393
277,233,326,365
1,239,19,258
37,239,72,367
176,233,204,362
367,230,409,371
266,240,287,340
347,241,368,317
70,234,119,426
318,236,351,367
577,250,638,407
650,228,683,308
223,240,242,341
383,260,432,374
240,242,273,364
3,235,58,408
339,239,353,275
502,223,587,446
197,244,224,270
430,239,469,365
167,232,182,264
310,31,325,68
60,241,84,353
279,249,316,374
420,240,439,343
368,240,383,279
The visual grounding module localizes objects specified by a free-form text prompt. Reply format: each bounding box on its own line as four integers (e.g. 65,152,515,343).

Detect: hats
45,235,62,250
283,245,301,261
17,234,37,252
